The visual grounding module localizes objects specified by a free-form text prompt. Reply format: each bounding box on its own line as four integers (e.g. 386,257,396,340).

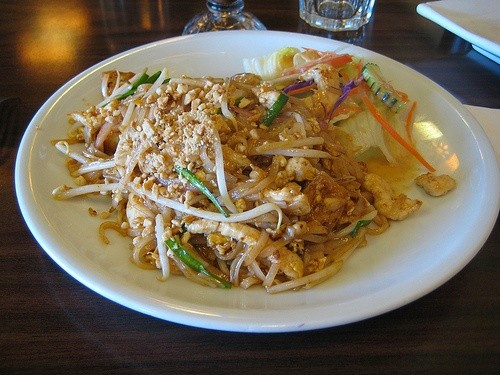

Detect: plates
416,0,500,66
12,30,500,334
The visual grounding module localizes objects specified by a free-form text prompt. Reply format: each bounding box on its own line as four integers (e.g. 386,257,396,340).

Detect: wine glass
182,1,267,40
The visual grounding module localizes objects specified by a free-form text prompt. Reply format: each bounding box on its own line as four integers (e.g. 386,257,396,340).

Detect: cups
299,0,375,33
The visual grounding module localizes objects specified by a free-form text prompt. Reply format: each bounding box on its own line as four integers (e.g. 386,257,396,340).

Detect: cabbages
244,48,436,180
